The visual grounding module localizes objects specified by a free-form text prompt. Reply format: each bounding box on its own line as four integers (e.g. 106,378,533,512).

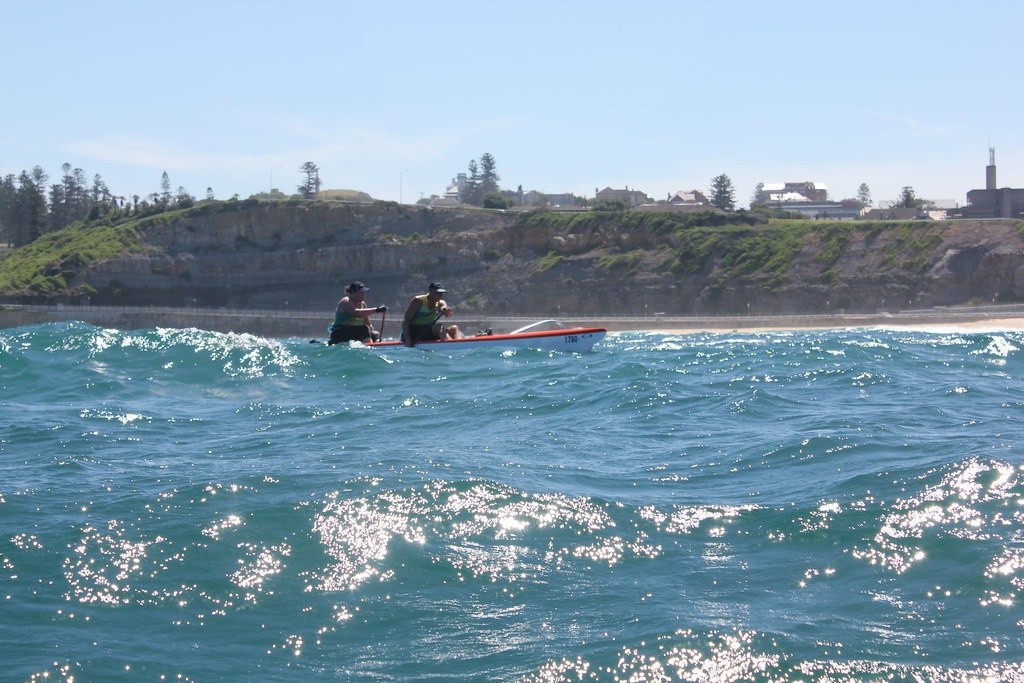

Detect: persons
327,281,388,346
400,282,462,348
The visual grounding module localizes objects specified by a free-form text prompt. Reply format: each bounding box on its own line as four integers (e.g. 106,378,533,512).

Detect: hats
429,281,447,292
350,281,370,292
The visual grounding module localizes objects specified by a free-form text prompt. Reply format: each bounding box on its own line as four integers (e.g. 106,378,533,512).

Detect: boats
362,324,608,355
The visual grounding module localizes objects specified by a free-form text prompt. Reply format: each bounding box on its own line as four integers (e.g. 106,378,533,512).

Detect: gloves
376,305,388,313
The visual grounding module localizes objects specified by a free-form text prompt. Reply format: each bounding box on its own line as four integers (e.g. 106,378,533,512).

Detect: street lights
399,166,408,205
